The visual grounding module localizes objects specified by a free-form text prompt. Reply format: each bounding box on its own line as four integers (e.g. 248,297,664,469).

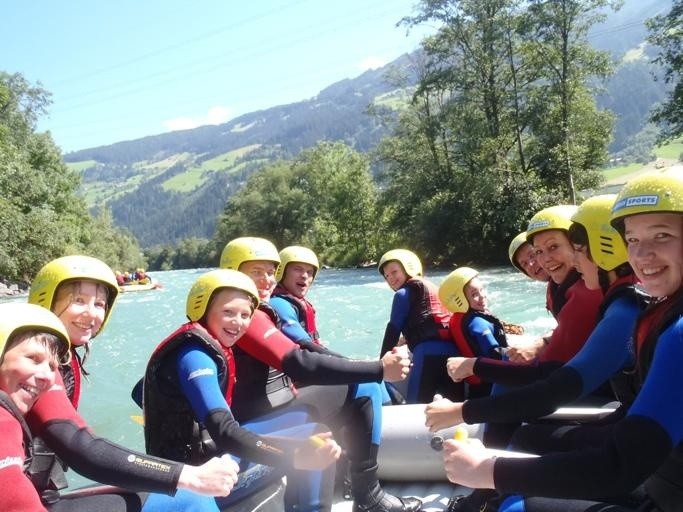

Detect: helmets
526,205,580,244
28,255,118,337
438,267,479,313
378,249,422,277
570,194,628,271
219,237,280,276
187,269,259,322
275,246,318,283
0,302,70,363
609,166,683,247
508,232,532,279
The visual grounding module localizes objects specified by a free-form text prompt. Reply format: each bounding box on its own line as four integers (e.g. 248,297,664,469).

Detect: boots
351,464,421,512
343,475,353,498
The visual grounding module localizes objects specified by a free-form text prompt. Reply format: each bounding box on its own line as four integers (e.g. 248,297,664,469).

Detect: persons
506,232,553,363
269,246,406,404
115,271,124,286
425,195,652,453
442,173,683,512
220,237,423,512
28,256,239,512
438,267,524,400
0,303,70,512
123,272,131,282
132,270,341,512
133,268,140,281
379,249,466,404
139,269,145,279
446,205,603,386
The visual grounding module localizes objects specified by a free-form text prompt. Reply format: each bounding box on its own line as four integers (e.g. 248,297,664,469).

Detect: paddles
132,414,327,451
